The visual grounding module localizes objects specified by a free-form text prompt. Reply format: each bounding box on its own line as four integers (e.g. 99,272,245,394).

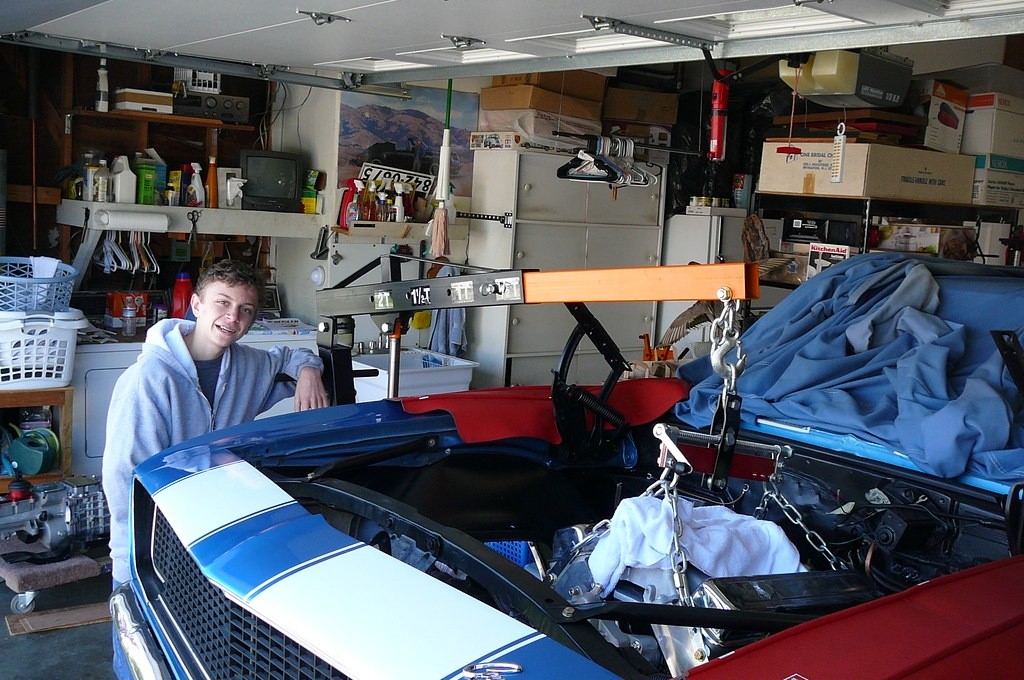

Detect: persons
100,259,329,680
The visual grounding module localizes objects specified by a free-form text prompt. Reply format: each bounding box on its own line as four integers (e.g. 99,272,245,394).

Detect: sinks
352,351,480,404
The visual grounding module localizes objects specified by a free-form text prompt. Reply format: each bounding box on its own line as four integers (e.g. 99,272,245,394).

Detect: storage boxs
104,314,146,328
104,292,148,316
602,88,678,125
603,122,672,162
477,109,601,145
491,69,606,100
755,79,1024,281
469,132,587,156
478,85,601,120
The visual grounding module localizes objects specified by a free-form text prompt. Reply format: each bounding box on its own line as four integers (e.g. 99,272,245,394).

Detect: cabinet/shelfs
0,385,74,501
1,40,269,307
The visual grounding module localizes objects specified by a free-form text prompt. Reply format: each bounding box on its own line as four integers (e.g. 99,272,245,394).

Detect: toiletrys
184,162,205,208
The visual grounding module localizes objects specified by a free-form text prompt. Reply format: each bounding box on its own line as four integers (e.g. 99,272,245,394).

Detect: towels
426,265,468,357
587,496,808,596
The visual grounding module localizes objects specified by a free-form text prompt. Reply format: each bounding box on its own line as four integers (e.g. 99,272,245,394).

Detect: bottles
93,160,109,203
172,271,194,319
82,153,99,201
164,182,175,206
386,199,394,222
121,296,136,337
110,155,137,204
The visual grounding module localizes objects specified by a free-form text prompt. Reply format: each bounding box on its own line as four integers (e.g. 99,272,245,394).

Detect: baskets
0,308,89,391
0,256,80,346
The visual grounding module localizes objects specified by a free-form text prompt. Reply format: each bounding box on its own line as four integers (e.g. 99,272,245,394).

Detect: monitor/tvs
235,149,302,213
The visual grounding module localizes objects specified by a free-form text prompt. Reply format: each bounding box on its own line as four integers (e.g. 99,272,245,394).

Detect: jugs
0,423,60,476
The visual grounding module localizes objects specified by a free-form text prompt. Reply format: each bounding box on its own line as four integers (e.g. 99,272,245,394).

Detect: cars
107,263,1024,680
355,142,462,178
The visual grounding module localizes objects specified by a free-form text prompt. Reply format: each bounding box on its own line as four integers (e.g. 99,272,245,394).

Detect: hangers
556,134,663,188
90,231,159,274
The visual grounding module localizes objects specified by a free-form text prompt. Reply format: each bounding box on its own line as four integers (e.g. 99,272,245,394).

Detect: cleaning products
172,272,194,319
339,178,413,227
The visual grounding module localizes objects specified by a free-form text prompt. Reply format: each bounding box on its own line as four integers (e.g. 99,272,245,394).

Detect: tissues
217,168,248,210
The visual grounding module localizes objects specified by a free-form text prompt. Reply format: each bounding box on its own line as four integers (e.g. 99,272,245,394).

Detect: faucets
378,332,394,349
